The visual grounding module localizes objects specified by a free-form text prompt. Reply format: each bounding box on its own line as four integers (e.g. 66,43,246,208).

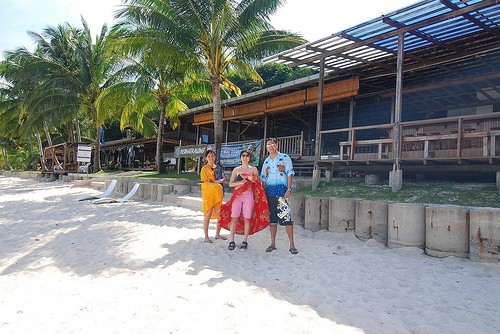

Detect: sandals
240,242,247,249
228,242,235,250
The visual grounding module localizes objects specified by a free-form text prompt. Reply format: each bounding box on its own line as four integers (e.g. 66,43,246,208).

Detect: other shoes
204,239,213,243
215,236,227,240
266,246,277,252
289,248,298,254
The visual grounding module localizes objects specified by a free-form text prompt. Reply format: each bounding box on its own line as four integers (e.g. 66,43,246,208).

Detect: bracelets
287,188,292,192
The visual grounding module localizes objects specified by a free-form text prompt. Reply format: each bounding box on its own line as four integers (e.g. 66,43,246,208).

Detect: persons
260,138,298,254
228,150,259,250
200,150,227,243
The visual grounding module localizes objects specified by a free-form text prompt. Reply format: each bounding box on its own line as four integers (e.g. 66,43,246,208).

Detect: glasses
241,155,249,157
266,142,274,146
